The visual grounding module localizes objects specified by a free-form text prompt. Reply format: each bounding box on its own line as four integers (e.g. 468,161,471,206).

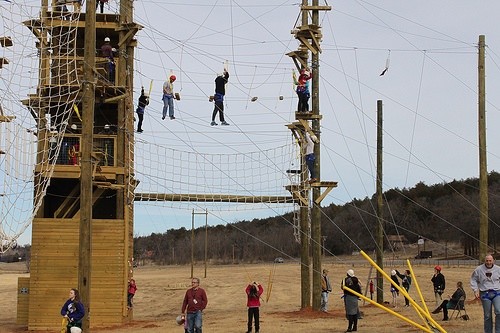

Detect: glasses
192,282,198,283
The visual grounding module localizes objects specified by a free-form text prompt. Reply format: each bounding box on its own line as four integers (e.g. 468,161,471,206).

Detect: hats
176,315,182,325
322,269,330,273
251,281,258,286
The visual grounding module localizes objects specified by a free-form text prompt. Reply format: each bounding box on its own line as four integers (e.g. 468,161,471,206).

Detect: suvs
274,258,284,264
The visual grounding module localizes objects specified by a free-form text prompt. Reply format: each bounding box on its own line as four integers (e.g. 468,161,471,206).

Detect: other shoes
310,178,317,183
162,115,165,119
171,116,174,120
222,121,229,125
211,121,218,126
137,129,143,132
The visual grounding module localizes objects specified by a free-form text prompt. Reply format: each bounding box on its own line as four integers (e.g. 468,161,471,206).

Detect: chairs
449,295,467,319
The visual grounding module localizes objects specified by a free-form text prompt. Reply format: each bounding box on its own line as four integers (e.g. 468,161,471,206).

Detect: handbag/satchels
61,317,69,333
462,314,469,320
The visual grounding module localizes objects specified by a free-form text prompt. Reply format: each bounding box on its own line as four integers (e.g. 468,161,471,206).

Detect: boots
432,306,441,314
347,327,352,331
352,326,357,331
442,314,448,321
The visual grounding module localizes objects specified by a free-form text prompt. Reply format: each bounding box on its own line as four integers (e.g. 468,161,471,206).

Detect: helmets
70,326,83,333
170,75,176,80
347,269,355,277
104,37,111,42
434,266,441,271
391,270,397,276
303,71,310,77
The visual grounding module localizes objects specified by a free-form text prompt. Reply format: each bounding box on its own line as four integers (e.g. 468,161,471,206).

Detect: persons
293,69,313,112
162,75,179,120
469,254,500,333
395,269,412,307
210,69,231,126
301,132,318,183
101,37,118,82
61,288,85,333
390,270,400,310
430,266,446,312
432,282,466,321
320,269,332,313
182,277,207,333
127,278,137,309
135,89,149,133
341,269,363,333
55,0,108,14
68,124,116,166
246,281,264,333
